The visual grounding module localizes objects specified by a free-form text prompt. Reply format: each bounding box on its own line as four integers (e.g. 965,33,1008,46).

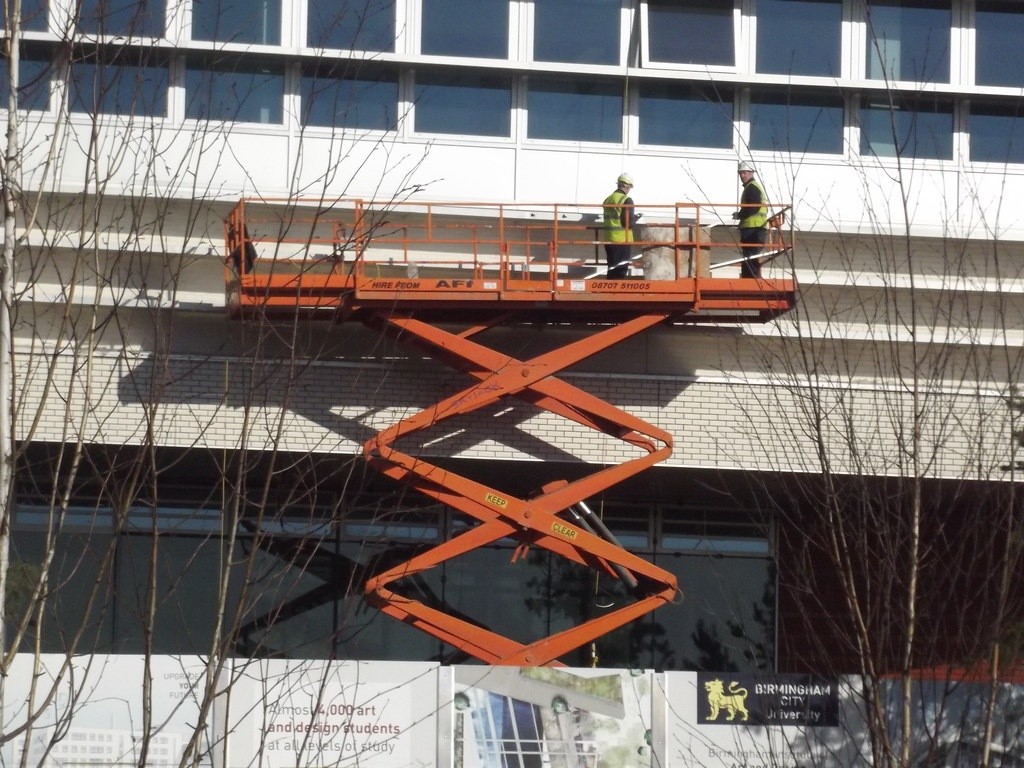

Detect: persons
602,172,644,280
732,160,768,279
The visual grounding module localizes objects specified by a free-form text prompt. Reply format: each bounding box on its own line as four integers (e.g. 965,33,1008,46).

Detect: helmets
618,174,633,188
737,162,755,172
